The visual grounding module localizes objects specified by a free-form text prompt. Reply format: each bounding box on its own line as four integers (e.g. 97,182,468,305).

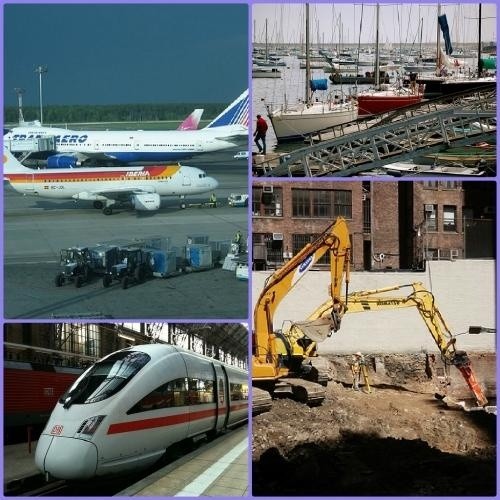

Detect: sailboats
252,3,497,145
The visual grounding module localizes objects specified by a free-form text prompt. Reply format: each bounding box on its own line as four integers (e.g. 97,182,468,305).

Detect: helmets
355,352,362,357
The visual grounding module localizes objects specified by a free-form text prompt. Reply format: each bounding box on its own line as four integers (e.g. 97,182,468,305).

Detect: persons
406,71,416,95
350,350,363,392
252,114,269,156
234,230,243,254
209,192,217,208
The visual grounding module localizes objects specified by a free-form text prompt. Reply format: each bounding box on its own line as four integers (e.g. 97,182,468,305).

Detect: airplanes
3,88,250,167
2,146,219,217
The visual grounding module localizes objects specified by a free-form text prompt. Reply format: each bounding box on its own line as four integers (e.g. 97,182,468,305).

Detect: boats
381,160,486,177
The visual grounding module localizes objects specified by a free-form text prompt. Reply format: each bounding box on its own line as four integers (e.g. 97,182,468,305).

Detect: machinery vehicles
55,245,153,290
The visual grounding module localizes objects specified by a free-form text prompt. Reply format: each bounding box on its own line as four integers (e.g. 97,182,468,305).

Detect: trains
34,342,249,483
